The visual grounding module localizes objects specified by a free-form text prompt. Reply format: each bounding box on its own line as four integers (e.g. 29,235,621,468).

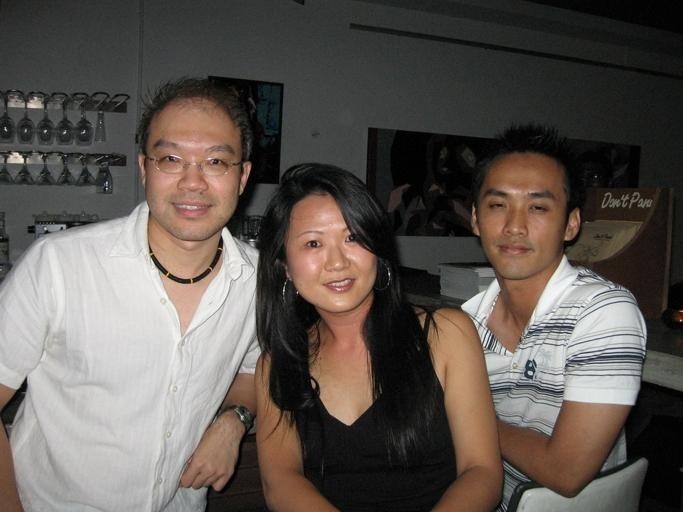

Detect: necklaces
145,231,225,283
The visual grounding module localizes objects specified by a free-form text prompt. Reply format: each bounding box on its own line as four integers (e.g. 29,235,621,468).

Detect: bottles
0,211,9,266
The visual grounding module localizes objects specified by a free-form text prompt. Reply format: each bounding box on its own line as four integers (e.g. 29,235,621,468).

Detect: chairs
506,458,651,512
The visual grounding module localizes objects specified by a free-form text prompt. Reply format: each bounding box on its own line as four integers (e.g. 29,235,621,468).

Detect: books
563,186,675,321
436,260,498,300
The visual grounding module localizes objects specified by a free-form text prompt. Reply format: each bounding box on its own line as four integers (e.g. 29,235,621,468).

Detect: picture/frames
207,76,284,184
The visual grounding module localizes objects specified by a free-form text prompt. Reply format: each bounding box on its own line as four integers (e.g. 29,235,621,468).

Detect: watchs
218,405,253,432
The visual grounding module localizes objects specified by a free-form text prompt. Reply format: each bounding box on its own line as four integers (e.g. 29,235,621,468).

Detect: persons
253,163,504,512
389,157,473,235
460,122,647,511
0,77,262,512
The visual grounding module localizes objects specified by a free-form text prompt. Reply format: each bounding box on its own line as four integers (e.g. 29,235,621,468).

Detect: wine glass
0,89,114,195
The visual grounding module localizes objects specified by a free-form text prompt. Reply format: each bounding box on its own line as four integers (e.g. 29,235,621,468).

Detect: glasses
144,153,242,174
